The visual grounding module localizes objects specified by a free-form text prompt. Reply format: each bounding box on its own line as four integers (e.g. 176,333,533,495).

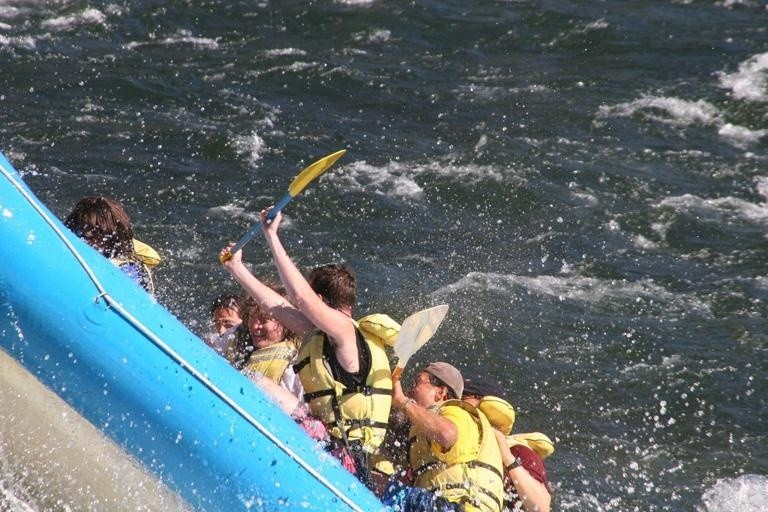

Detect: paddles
219,149,347,262
391,305,448,386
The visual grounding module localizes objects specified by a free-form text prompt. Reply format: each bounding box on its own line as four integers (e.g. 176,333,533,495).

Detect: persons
64,196,163,301
213,207,555,511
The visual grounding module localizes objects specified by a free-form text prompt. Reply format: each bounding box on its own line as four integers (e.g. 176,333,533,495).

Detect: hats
422,360,466,401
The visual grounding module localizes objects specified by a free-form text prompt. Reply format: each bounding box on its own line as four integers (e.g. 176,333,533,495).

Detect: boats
0,146,392,512
